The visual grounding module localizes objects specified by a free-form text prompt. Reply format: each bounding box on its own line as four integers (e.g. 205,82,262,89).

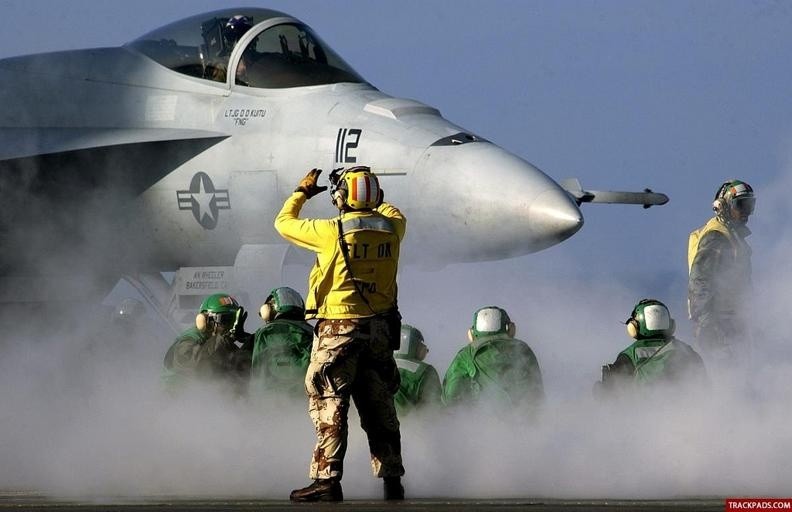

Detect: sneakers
384,482,404,500
291,478,343,501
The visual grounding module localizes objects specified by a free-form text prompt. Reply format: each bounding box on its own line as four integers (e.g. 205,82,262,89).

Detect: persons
248,287,315,401
155,292,253,409
591,298,711,415
393,325,445,422
438,307,547,414
207,14,260,89
273,166,407,504
686,178,757,384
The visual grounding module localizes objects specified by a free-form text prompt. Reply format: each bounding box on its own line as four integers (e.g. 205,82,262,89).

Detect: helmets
712,179,753,223
258,286,305,321
393,324,427,360
196,293,240,334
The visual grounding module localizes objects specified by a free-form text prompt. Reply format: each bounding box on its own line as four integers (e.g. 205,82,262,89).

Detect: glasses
731,198,755,213
210,313,234,326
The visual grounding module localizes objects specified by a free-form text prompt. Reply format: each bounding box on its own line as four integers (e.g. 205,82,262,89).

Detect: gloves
295,168,327,199
226,306,247,341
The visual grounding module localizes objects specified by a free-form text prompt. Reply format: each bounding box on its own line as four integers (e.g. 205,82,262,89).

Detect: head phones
713,183,731,216
225,15,253,41
196,310,209,333
259,300,274,321
416,329,429,361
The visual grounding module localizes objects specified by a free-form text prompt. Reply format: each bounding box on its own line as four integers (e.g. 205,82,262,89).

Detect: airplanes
0,6,669,335
